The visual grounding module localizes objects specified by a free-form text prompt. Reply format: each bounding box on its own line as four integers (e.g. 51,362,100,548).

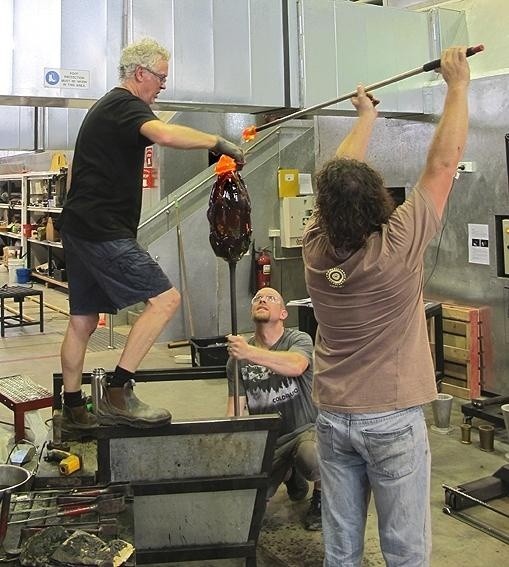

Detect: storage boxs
188,334,230,368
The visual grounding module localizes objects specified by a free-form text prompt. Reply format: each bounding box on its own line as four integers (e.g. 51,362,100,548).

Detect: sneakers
61,404,97,442
304,489,322,531
96,375,172,429
284,467,309,501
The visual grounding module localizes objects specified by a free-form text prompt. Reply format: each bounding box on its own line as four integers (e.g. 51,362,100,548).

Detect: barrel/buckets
7,259,25,284
14,268,32,284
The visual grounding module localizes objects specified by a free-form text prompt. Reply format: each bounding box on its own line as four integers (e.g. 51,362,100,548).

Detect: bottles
91,368,108,415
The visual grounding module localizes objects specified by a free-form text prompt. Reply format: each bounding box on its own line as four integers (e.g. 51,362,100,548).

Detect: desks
1,285,44,336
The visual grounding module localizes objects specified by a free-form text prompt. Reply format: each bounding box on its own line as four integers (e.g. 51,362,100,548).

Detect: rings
229,348,232,353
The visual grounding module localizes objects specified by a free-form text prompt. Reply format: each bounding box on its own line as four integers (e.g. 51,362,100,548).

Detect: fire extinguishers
255,245,272,294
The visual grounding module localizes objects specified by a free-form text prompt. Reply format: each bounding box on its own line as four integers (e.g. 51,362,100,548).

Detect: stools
0,374,54,443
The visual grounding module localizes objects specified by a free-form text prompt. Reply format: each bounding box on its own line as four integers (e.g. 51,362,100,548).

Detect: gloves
208,135,244,171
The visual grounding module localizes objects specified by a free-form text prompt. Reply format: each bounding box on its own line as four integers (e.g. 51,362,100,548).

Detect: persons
57,40,244,434
225,287,322,531
302,46,471,567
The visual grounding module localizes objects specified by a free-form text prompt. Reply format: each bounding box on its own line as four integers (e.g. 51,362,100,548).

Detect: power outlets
457,161,472,173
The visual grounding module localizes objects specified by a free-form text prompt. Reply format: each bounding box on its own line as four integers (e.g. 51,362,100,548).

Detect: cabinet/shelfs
297,296,445,394
0,171,67,290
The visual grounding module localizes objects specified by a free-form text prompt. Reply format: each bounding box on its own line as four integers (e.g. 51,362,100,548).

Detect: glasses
141,66,167,86
252,293,284,306
